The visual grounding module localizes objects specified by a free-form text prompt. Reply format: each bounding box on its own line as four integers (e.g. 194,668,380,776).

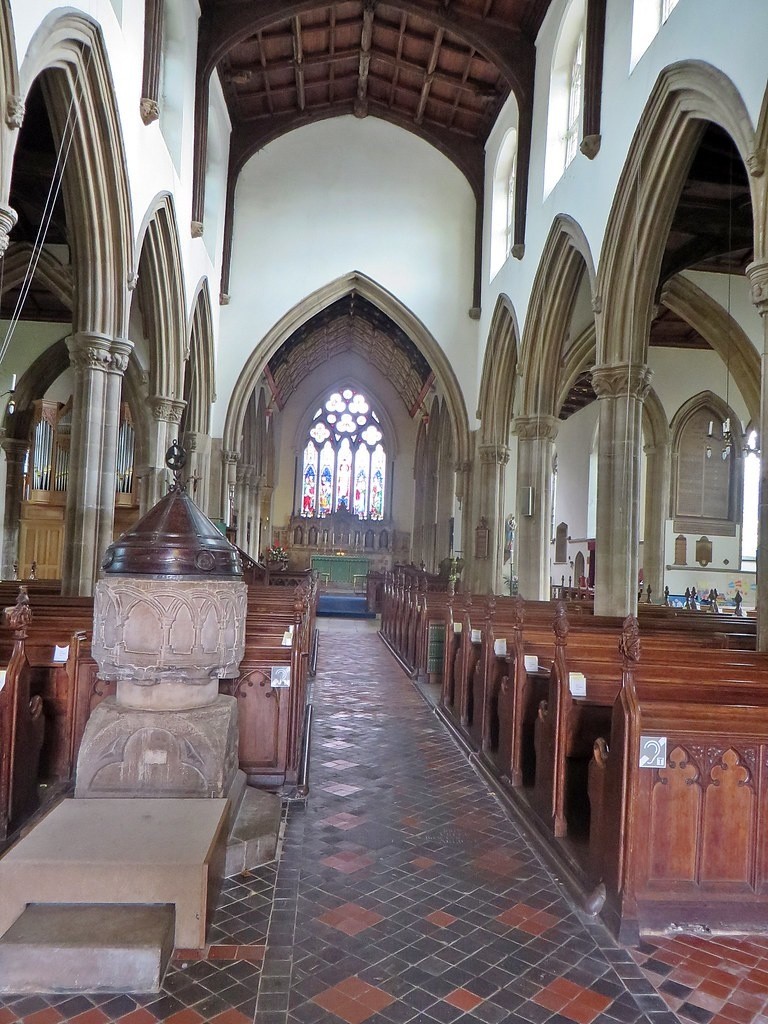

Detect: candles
333,533,334,545
363,534,366,546
317,532,319,545
324,532,327,545
11,374,17,391
355,534,358,546
349,534,351,546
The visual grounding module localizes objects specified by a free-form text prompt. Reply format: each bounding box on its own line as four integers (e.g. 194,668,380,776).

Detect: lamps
706,251,762,461
0,391,15,414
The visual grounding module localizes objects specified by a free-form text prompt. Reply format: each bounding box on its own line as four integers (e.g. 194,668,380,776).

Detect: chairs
371,563,768,946
0,548,320,862
